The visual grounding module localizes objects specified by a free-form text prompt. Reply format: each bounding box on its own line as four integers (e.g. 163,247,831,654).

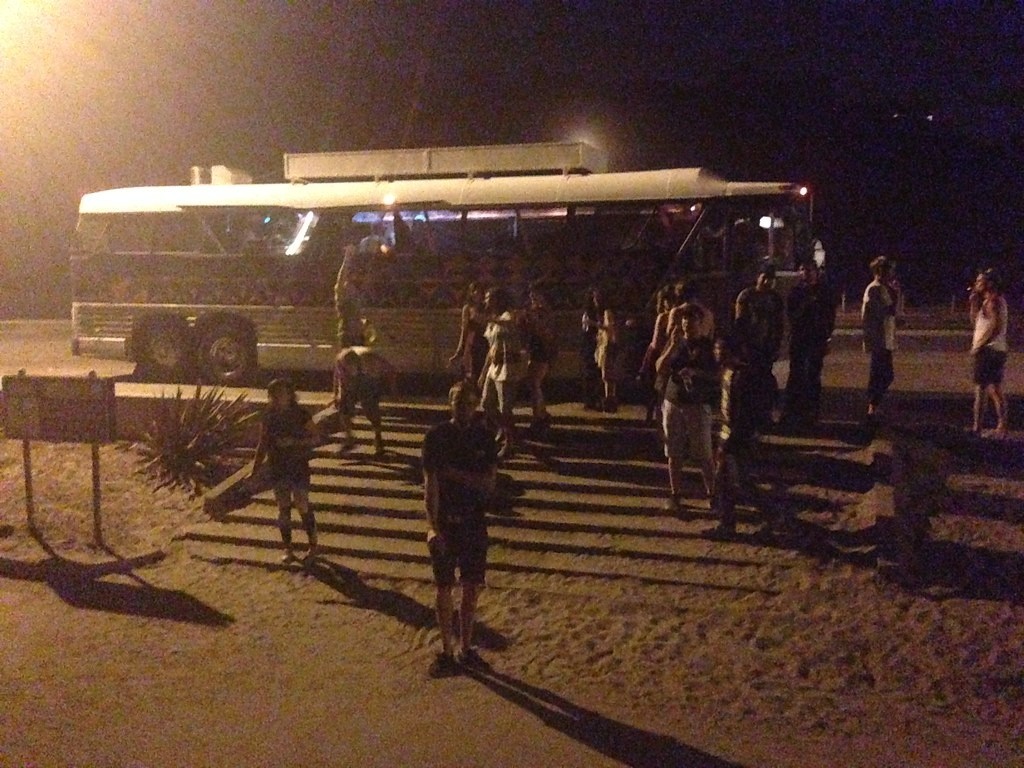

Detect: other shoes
301,547,318,566
457,647,479,663
281,548,295,561
701,524,736,541
866,411,888,423
371,445,384,457
429,650,455,672
496,441,516,457
662,494,683,510
337,438,357,453
754,525,791,542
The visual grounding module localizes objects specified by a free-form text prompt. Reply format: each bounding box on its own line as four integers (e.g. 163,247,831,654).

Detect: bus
65,169,816,398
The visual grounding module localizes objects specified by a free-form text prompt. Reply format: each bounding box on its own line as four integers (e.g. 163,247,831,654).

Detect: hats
675,304,705,321
977,267,1002,290
757,262,778,277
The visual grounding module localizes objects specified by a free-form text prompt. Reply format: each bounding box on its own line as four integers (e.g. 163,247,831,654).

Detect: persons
335,248,403,451
449,269,784,537
240,378,321,562
861,255,903,421
967,264,1008,440
420,379,498,677
787,258,834,413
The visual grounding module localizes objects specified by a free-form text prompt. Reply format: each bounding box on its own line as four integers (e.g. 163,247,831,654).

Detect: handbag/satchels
651,366,684,405
